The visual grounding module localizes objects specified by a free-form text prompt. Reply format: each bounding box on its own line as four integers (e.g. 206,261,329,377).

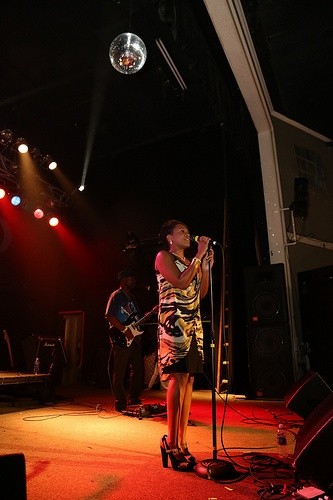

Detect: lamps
0,128,60,226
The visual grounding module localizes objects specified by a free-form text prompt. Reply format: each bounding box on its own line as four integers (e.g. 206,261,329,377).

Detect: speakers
283,369,333,475
245,284,295,401
0,453,28,500
30,337,62,374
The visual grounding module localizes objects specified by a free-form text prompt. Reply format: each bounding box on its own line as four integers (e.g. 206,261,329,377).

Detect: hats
117,270,134,280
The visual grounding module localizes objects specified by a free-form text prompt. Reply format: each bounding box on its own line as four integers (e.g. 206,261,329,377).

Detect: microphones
191,235,218,245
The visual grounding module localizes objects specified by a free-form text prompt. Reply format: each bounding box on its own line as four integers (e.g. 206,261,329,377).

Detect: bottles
33,358,40,375
277,424,288,459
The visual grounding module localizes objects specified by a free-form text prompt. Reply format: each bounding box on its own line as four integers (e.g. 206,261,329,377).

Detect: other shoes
114,400,125,411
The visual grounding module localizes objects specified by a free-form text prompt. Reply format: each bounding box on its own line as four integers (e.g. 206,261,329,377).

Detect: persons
154,219,215,472
104,270,145,413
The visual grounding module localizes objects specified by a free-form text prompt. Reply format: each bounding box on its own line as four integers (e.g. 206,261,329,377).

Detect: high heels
160,435,196,471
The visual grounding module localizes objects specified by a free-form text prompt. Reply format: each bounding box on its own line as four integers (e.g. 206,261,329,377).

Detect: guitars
107,303,159,349
